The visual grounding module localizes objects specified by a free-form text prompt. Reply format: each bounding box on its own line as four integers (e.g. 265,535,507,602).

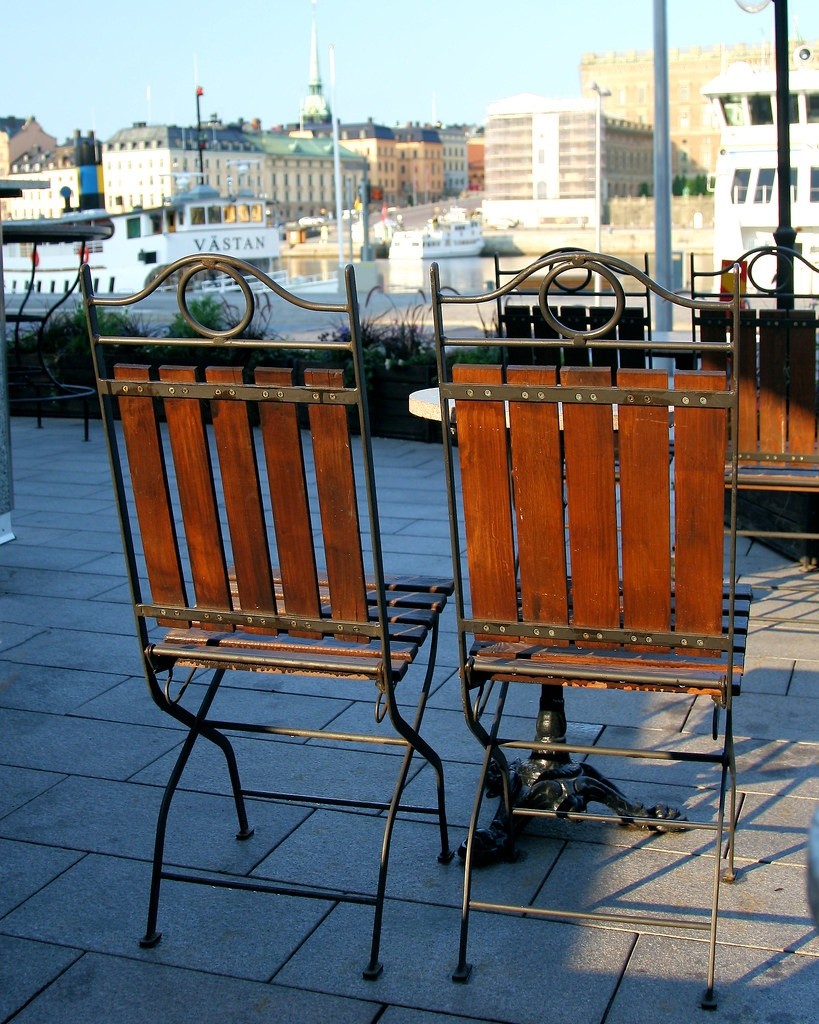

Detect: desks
3,225,112,442
410,387,690,860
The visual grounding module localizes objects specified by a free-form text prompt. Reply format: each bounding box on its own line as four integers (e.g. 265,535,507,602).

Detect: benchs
516,275,611,293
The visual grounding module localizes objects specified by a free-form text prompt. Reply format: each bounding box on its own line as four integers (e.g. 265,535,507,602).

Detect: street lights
589,82,611,308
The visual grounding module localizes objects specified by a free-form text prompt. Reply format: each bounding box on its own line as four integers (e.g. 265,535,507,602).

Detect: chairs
78,252,454,981
428,261,752,1009
494,248,660,577
691,246,819,628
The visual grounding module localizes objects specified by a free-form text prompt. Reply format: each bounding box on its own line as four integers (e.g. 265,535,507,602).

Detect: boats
388,206,485,259
2,85,338,315
702,46,819,312
